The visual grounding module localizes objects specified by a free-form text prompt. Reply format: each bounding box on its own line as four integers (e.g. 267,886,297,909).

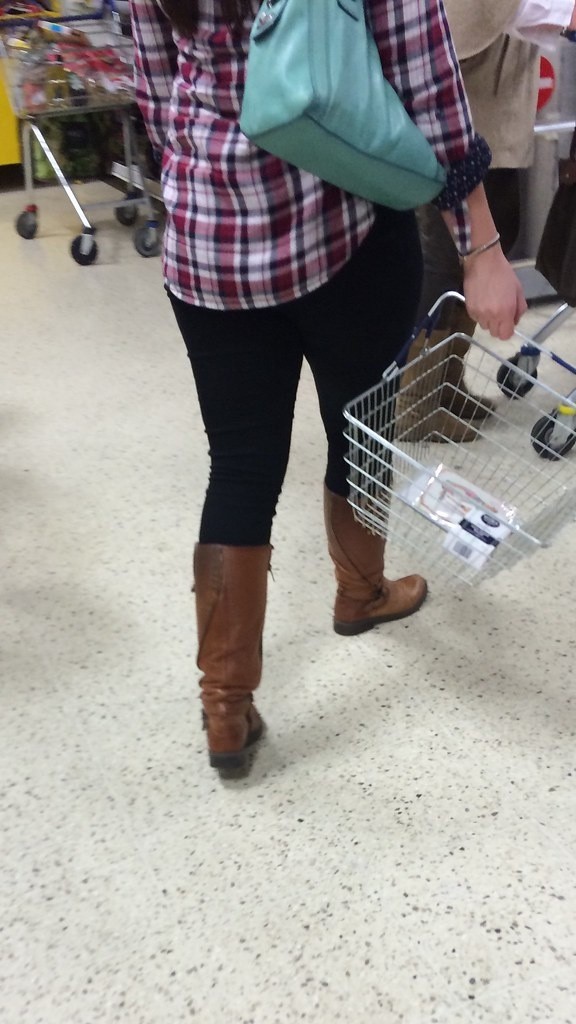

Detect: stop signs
537,56,555,113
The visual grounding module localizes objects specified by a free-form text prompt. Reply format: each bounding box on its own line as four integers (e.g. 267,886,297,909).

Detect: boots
440,306,496,419
192,541,274,767
394,326,481,442
324,474,428,636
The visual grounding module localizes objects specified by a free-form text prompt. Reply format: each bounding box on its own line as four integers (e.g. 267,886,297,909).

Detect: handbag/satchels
238,0,446,212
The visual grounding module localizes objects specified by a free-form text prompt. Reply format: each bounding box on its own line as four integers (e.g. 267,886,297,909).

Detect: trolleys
2,10,164,266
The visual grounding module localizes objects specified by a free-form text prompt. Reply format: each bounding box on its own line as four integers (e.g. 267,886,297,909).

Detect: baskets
346,330,576,586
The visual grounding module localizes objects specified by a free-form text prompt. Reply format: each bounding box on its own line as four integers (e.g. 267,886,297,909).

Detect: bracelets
458,233,501,261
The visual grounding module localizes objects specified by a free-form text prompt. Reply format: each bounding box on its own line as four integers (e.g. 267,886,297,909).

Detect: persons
371,0,539,443
126,0,528,775
